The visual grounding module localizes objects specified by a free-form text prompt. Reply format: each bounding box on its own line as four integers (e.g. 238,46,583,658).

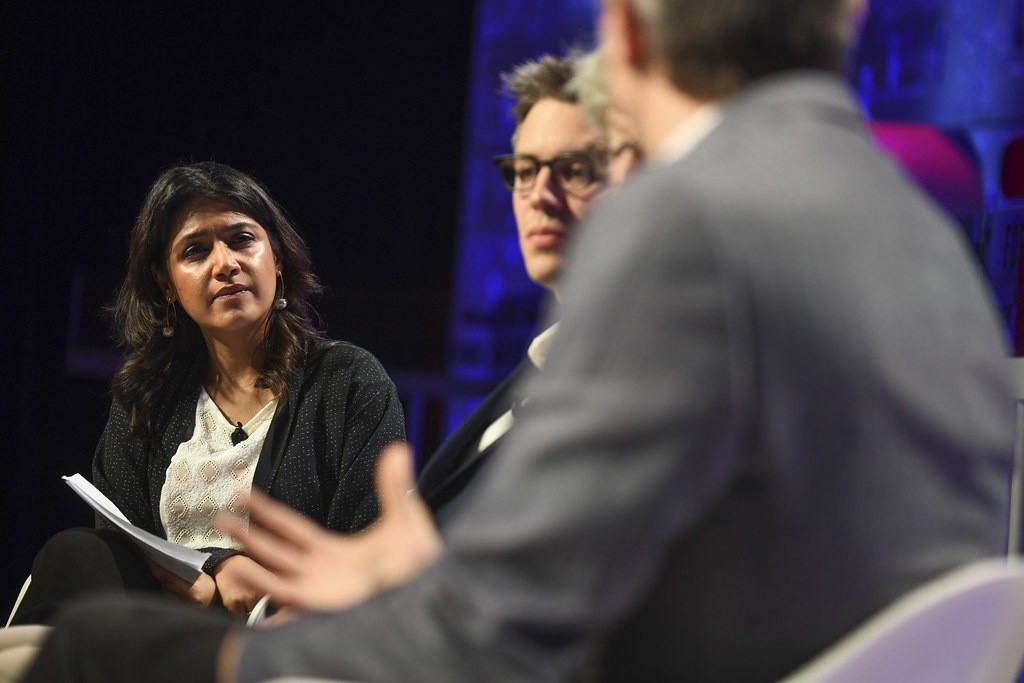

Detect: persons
30,158,409,660
10,0,1021,683
569,29,674,195
246,42,620,682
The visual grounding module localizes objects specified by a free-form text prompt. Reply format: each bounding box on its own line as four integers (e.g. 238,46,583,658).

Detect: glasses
493,152,610,193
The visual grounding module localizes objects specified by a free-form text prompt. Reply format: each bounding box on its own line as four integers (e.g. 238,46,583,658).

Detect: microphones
230,421,248,447
